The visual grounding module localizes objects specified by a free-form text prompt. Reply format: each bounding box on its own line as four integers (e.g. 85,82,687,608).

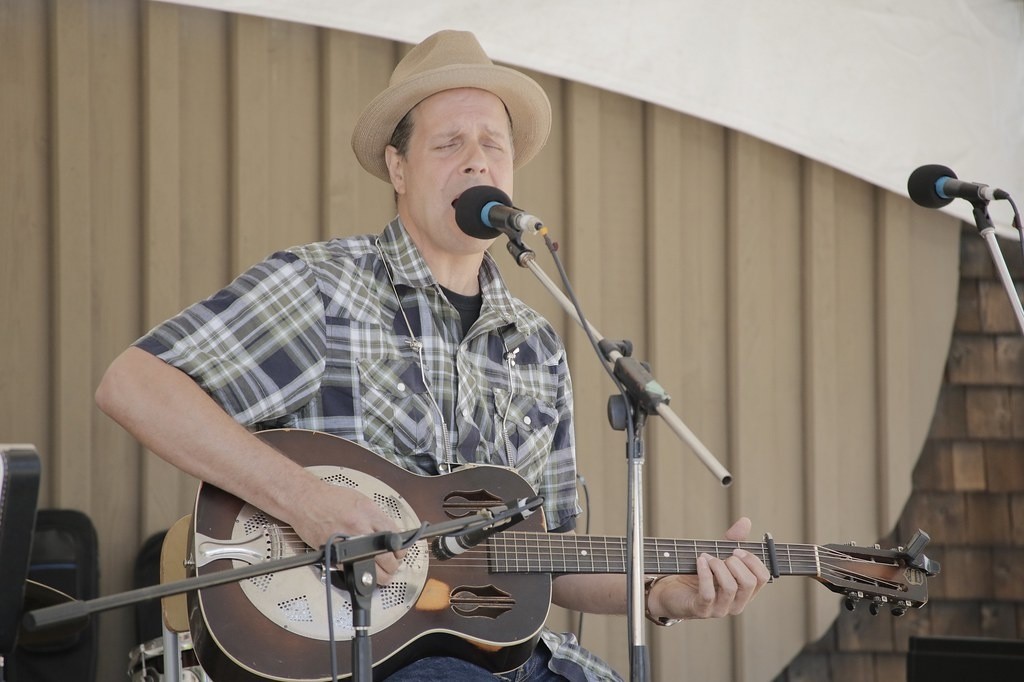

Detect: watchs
644,574,686,629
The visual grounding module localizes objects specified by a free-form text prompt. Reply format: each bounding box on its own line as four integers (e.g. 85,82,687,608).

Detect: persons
93,28,770,682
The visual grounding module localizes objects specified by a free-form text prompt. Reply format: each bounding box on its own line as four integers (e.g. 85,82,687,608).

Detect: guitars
184,428,932,681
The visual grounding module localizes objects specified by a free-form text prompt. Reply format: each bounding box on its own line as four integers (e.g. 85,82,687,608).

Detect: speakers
132,529,199,671
0,507,97,682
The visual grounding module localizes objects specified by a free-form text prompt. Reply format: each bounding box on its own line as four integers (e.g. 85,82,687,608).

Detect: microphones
455,185,544,240
908,164,1009,208
431,496,545,560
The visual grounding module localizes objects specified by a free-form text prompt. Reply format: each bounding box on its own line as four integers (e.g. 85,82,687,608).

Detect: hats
351,30,552,183
